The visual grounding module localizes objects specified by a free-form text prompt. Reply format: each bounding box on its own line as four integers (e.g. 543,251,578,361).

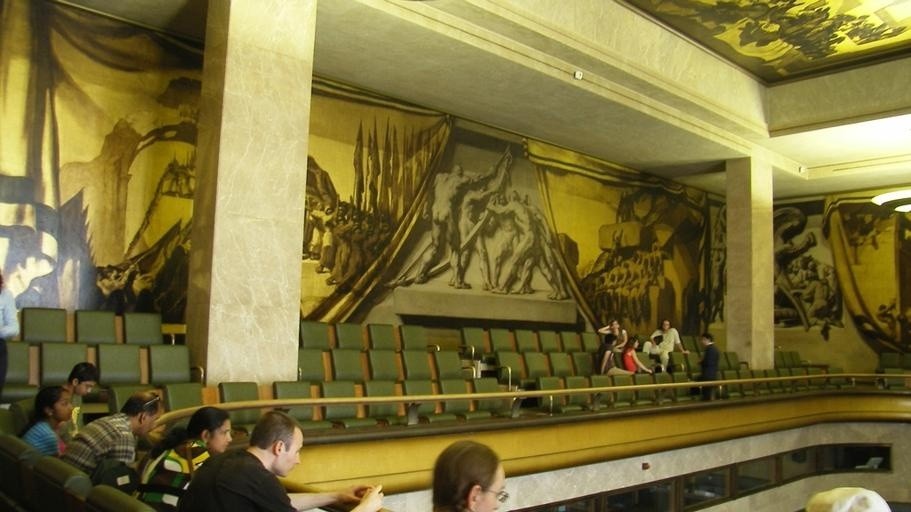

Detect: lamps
868,187,911,215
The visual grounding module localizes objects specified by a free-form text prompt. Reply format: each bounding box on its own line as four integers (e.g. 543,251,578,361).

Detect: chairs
297,319,911,431
0,307,333,511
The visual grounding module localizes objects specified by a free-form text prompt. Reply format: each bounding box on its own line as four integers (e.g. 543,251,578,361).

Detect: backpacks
90,449,186,497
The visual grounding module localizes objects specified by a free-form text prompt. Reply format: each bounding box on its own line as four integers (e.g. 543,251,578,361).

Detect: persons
596,319,721,400
804,487,893,512
130,407,233,512
183,411,385,512
18,386,74,459
433,440,511,512
58,362,98,439
775,258,846,335
96,264,155,298
62,392,160,479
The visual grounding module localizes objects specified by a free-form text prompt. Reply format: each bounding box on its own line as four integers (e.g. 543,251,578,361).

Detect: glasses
143,393,160,409
483,489,509,503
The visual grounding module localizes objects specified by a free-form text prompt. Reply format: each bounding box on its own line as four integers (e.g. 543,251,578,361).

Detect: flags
353,115,441,220
